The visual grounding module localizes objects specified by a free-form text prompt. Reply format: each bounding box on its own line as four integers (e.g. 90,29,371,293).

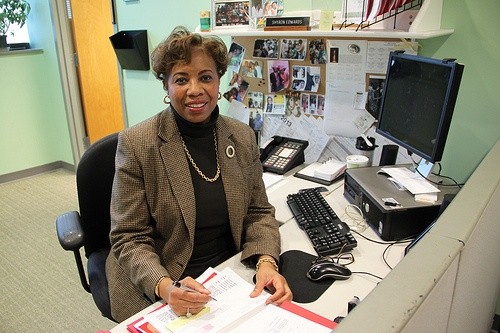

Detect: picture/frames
210,0,252,33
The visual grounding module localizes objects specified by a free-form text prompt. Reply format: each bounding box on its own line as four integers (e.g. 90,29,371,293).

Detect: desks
110,163,413,333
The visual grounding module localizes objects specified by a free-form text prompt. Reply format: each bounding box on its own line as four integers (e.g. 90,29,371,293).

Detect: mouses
306,262,352,281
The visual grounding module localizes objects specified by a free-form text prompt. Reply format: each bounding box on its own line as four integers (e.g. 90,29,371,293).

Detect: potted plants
0,0,31,48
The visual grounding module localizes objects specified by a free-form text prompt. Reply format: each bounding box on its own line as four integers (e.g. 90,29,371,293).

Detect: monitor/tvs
375,52,465,204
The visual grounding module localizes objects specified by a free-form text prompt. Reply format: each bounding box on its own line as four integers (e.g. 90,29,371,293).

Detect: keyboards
286,188,358,258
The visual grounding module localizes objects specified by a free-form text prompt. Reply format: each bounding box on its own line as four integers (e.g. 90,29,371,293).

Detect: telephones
259,135,309,175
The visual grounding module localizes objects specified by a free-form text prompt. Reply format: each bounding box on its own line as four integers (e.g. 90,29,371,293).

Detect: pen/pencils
172,281,217,301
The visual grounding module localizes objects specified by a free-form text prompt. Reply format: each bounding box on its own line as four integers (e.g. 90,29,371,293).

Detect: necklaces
180,127,220,182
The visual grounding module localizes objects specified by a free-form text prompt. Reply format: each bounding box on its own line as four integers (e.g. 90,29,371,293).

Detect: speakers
379,145,399,166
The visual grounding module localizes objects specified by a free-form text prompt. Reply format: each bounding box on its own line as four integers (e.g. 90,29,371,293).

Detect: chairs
56,132,121,324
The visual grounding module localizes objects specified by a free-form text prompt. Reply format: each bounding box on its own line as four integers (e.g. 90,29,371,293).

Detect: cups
346,154,370,170
354,136,378,168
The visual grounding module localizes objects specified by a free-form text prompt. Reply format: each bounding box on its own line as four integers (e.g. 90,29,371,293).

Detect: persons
100,25,294,324
367,81,385,112
215,2,324,128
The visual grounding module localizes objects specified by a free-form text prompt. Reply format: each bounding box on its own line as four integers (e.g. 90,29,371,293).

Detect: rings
186,308,191,317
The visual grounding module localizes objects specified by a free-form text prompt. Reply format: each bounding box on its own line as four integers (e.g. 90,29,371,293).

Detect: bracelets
155,276,164,297
255,257,278,272
